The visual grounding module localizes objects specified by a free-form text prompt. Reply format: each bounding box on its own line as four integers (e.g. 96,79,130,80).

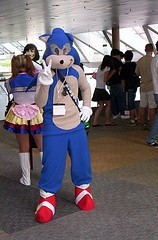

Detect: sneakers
113,114,130,120
149,140,158,148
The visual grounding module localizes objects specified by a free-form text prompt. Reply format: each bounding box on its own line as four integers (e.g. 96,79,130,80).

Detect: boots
19,152,32,186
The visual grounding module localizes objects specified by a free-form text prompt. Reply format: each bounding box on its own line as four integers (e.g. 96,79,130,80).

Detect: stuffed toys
33,28,94,222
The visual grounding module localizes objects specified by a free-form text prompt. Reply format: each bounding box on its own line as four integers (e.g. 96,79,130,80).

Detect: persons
147,40,158,148
107,49,130,120
121,50,140,127
135,43,156,129
4,53,45,187
88,54,117,127
4,45,48,170
117,52,129,111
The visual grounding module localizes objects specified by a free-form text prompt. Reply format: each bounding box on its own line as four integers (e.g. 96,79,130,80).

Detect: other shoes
75,183,94,211
125,120,139,126
35,193,57,223
92,123,103,127
104,122,118,127
